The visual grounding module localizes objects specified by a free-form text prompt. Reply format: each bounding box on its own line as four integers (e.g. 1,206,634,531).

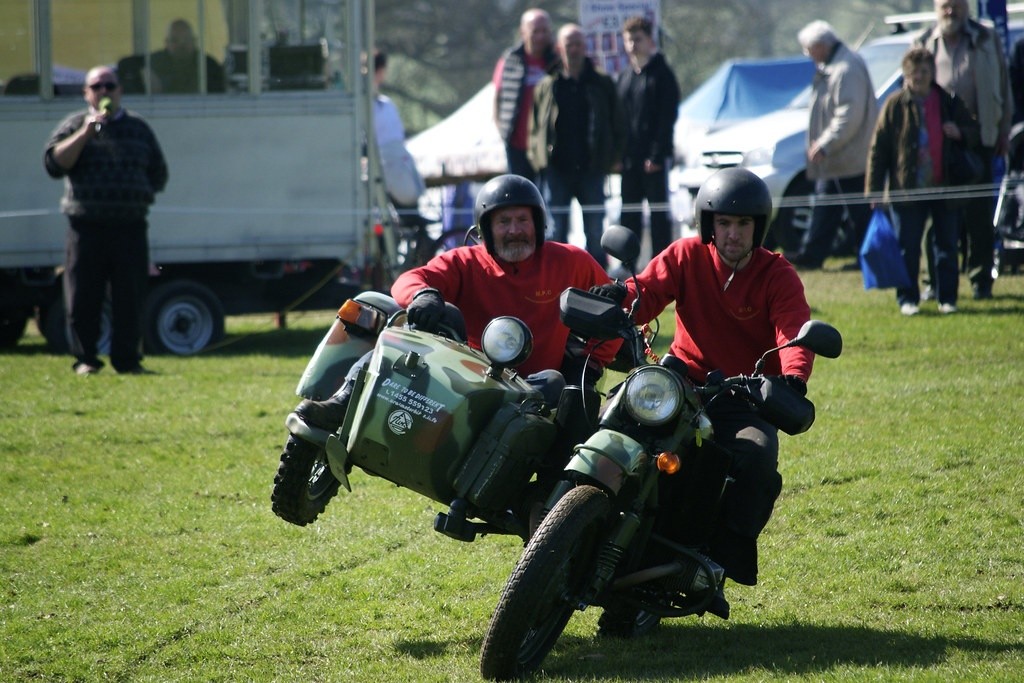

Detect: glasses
89,82,116,93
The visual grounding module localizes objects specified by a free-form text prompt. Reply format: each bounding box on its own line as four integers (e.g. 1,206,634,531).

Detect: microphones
95,97,113,132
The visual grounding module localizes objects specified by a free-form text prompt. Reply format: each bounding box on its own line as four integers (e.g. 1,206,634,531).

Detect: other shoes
73,359,98,373
842,264,859,270
110,354,145,377
922,282,936,301
971,277,994,299
784,256,823,269
901,301,919,315
939,303,957,314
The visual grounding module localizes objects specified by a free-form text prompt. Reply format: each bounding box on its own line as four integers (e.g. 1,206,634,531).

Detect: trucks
1,1,377,368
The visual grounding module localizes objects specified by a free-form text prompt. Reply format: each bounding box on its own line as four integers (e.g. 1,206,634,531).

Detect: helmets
696,167,772,244
475,174,545,261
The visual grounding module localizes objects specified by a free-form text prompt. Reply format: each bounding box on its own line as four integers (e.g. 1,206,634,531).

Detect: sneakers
608,262,667,284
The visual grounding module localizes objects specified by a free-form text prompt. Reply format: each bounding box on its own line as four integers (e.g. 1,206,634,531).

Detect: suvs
687,2,1024,262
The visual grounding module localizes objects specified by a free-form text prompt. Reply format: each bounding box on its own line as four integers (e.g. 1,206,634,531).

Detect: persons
391,169,626,384
361,48,425,207
43,65,168,376
618,17,680,281
863,50,977,316
492,8,559,182
526,24,617,270
148,19,226,93
789,20,876,273
910,0,1011,300
592,167,815,587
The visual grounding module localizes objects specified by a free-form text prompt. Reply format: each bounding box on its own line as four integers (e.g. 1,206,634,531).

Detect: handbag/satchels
860,207,908,288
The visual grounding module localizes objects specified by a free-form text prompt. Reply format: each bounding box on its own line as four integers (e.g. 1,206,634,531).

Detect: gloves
406,287,448,332
559,354,603,386
588,284,627,306
775,373,808,423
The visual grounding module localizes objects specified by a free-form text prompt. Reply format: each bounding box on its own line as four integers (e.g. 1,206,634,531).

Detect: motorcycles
268,225,843,681
